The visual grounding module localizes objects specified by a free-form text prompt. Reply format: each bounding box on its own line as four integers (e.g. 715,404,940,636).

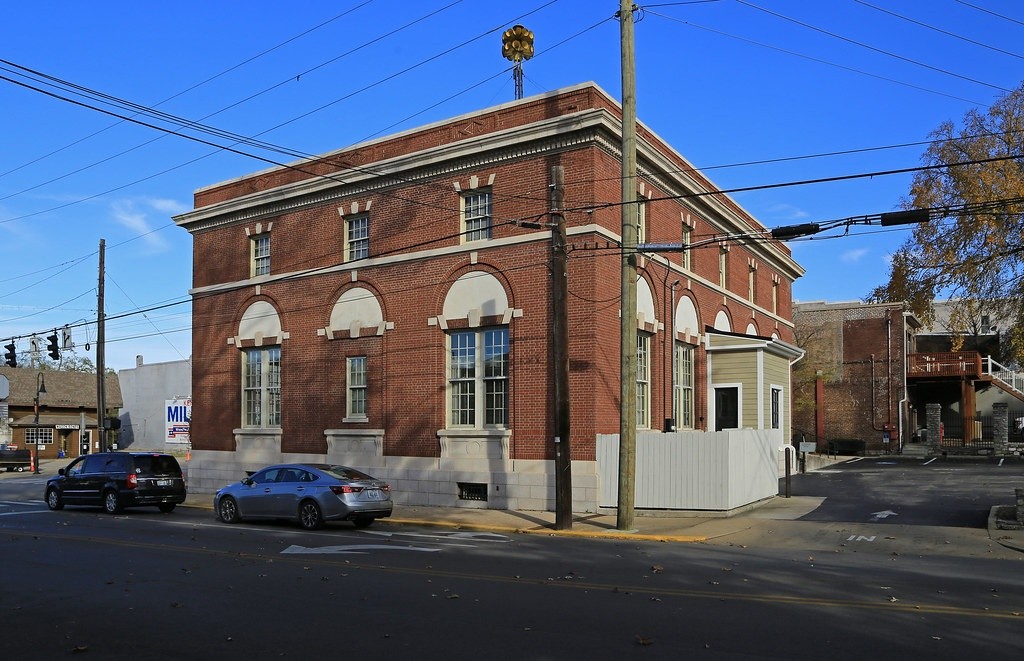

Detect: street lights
33,370,46,474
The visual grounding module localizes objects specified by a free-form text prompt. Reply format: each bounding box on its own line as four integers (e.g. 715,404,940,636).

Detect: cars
213,463,393,531
44,451,187,515
